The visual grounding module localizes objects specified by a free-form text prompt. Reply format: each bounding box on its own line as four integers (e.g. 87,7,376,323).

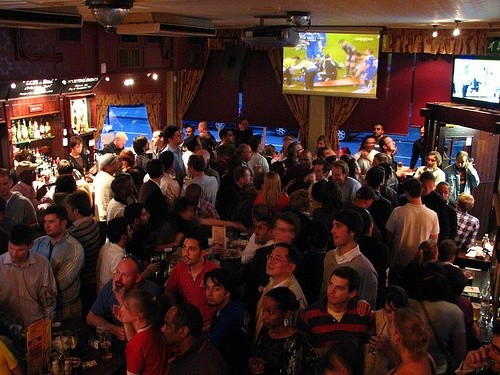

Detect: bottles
11,117,60,185
481,233,489,245
52,360,59,374
65,361,71,374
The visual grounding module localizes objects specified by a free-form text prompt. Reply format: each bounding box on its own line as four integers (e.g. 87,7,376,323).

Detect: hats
16,161,43,175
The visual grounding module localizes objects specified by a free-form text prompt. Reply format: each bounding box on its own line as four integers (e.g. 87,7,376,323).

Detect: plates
227,249,243,259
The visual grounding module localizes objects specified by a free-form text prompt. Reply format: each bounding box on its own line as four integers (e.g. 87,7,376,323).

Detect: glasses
489,341,500,355
267,255,293,264
122,254,144,272
428,159,436,162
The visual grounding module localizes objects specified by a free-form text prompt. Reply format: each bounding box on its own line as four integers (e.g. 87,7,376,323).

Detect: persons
462,64,496,100
0,116,500,375
283,32,378,94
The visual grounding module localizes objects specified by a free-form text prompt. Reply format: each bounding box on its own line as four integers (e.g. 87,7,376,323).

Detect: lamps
432,20,461,38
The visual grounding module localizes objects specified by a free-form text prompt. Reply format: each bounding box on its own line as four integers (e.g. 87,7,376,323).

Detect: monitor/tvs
451,55,500,111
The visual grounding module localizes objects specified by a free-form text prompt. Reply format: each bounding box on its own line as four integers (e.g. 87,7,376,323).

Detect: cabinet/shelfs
0,90,98,176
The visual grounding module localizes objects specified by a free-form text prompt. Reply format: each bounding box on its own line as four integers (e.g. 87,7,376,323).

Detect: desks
18,317,126,375
454,240,493,344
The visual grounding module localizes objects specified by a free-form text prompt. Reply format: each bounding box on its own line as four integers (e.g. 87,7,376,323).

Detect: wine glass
150,254,160,280
60,336,71,360
98,331,112,359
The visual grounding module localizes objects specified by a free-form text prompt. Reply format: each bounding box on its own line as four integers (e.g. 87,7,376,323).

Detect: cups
398,162,403,173
473,302,481,321
164,248,172,264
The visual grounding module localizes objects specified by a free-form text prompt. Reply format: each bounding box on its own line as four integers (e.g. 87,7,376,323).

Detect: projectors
241,25,299,48
